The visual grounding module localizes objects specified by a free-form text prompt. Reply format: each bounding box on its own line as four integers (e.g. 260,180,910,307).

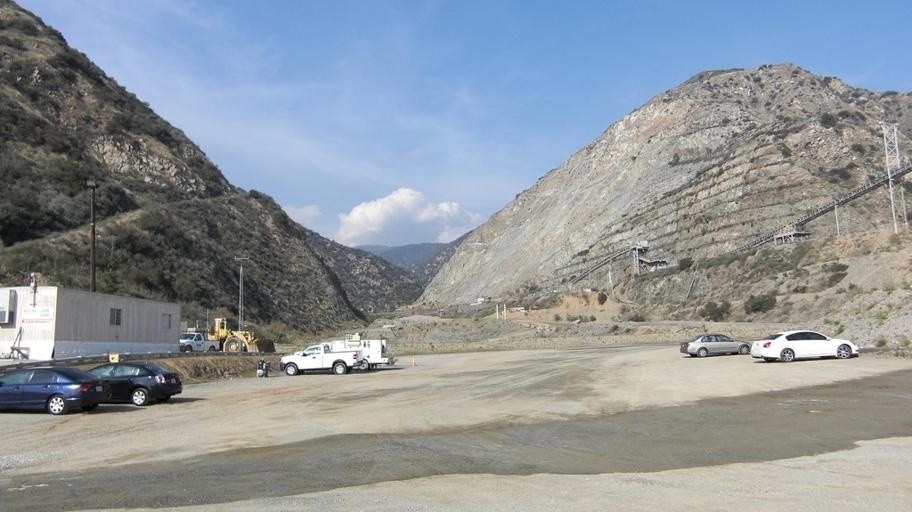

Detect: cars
679,333,751,358
85,360,184,407
750,329,860,362
1,365,112,415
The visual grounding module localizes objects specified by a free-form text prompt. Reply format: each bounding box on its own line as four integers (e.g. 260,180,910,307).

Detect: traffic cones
411,358,417,367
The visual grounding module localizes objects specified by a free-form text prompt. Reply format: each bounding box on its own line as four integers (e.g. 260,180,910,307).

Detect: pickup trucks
180,331,221,353
280,344,363,376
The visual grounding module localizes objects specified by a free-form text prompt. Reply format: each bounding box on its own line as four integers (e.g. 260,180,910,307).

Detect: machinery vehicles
207,318,277,355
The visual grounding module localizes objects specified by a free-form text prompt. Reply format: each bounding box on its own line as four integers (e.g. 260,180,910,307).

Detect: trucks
293,333,391,372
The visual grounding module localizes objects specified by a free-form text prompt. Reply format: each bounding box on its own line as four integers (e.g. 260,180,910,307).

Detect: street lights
87,179,101,291
